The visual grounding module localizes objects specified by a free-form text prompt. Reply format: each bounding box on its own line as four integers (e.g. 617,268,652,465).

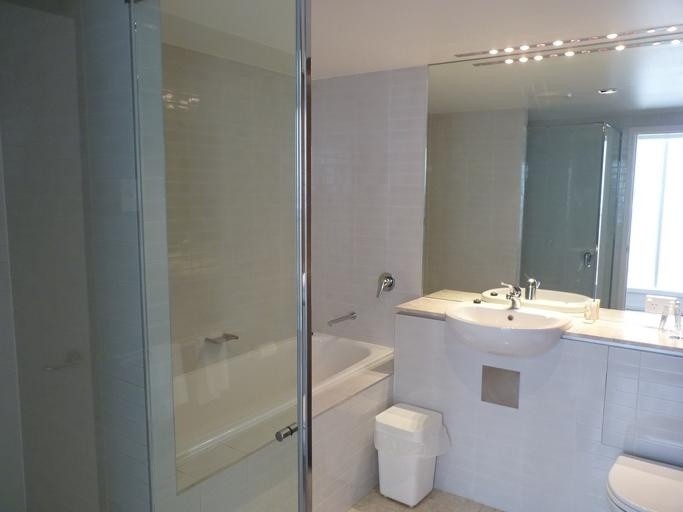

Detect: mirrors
421,30,683,318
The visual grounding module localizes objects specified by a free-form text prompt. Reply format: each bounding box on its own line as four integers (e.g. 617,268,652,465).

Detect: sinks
445,299,574,357
481,282,600,316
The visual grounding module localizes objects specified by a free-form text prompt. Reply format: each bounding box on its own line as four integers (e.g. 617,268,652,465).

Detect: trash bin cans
374,403,443,508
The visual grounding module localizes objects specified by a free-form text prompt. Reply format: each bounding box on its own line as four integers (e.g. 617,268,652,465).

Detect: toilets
607,450,682,512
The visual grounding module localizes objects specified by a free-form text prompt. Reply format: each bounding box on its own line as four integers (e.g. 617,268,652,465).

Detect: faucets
525,277,542,301
498,283,523,309
326,311,357,327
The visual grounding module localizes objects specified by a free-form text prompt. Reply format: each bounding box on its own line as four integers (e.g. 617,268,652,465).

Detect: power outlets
644,295,678,316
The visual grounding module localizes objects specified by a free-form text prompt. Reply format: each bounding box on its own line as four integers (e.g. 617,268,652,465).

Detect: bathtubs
173,328,393,495
